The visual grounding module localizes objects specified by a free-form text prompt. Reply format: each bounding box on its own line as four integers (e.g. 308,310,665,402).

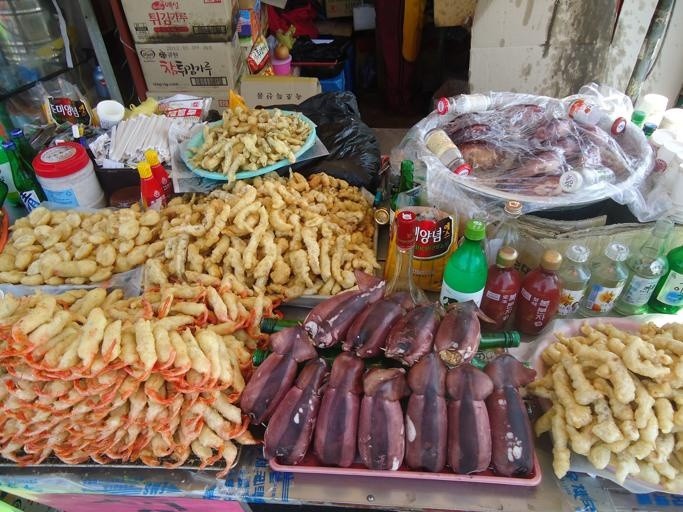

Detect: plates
179,108,317,181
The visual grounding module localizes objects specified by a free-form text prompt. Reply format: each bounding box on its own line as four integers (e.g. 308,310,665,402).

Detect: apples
274,43,289,60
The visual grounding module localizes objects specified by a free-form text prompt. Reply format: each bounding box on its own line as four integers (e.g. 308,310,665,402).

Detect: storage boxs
120,0,376,118
419,155,682,284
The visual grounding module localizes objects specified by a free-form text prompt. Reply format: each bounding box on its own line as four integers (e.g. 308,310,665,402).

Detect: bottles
253,316,299,368
477,247,521,333
438,220,488,309
555,244,593,319
511,250,561,338
0,124,107,212
565,96,645,135
390,211,430,310
647,243,683,314
423,91,488,178
613,218,676,317
485,200,525,264
137,150,174,209
396,157,419,203
575,243,628,318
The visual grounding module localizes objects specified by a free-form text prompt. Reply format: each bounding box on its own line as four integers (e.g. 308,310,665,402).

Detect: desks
0,129,683,512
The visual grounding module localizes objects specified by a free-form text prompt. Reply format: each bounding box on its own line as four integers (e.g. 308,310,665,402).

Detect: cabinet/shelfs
0,0,124,145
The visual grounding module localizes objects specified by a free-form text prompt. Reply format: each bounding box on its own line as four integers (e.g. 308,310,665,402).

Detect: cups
96,100,126,129
271,54,292,76
639,93,683,228
